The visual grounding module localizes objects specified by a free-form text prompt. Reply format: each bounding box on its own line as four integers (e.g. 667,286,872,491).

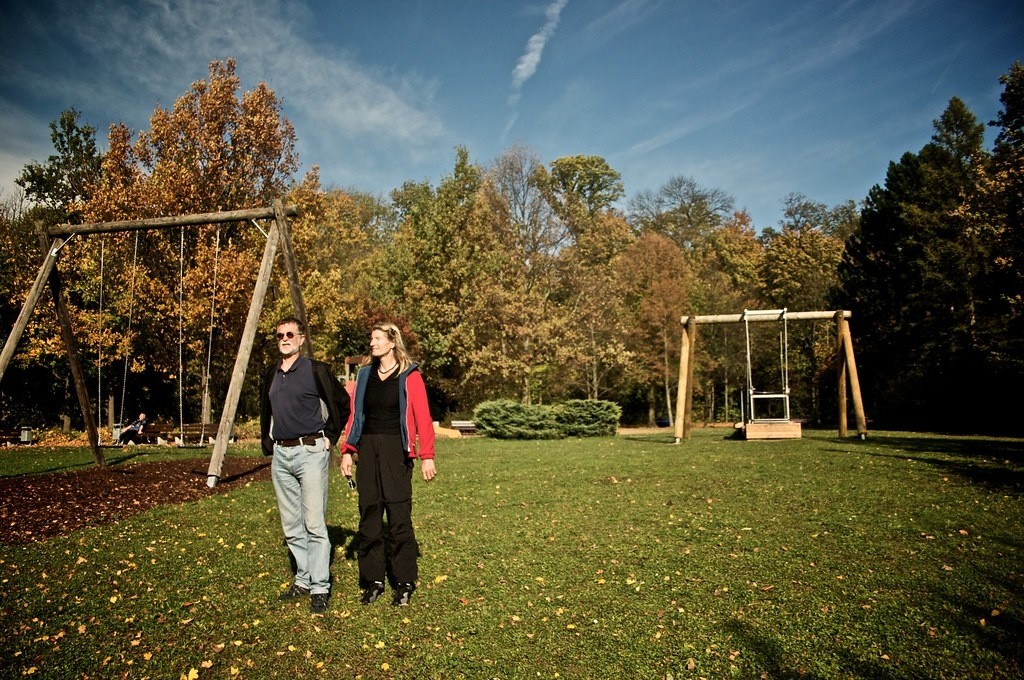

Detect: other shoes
278,585,310,602
359,581,385,605
311,593,331,613
391,582,417,607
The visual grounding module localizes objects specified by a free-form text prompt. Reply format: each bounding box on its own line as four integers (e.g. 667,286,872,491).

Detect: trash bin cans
20,426,32,442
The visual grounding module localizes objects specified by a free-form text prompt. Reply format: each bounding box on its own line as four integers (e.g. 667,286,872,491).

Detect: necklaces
378,362,397,375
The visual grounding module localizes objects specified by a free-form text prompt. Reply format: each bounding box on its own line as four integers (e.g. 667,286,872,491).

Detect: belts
274,432,323,447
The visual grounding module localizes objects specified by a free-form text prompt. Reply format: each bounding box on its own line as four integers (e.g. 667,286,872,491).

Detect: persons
260,316,350,608
336,321,437,605
113,412,148,447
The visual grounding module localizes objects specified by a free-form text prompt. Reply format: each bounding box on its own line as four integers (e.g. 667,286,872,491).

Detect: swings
93,231,139,449
173,226,221,451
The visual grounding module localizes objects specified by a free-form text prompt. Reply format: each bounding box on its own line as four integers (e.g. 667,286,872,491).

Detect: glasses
347,477,357,490
276,332,303,340
